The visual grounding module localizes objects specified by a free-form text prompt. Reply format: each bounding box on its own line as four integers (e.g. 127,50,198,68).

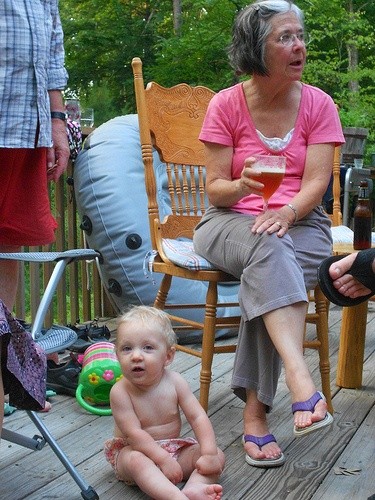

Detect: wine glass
251,155,286,214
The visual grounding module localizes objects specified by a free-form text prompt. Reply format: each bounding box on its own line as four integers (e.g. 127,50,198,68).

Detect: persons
0,0,70,318
317,248,375,307
192,0,346,468
104,305,225,499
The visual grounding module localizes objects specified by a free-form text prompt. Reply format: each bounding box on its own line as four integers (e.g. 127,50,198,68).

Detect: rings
274,221,281,227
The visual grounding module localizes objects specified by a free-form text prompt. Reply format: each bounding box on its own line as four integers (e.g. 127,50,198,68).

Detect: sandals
64,322,111,353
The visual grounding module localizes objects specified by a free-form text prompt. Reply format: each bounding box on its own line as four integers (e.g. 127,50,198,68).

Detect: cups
354,159,363,168
81,108,94,127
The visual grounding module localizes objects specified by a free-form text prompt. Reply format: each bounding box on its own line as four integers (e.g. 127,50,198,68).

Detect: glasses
265,33,312,47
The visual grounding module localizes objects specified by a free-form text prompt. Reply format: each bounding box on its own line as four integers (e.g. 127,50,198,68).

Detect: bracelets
51,111,66,120
284,203,297,225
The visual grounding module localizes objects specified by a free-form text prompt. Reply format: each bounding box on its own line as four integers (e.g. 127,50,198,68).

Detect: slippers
317,248,375,307
242,432,285,468
292,391,333,435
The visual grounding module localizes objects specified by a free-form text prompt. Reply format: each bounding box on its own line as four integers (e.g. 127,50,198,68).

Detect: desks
330,241,375,389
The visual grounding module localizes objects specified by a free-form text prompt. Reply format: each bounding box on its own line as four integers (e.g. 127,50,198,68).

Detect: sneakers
46,359,83,397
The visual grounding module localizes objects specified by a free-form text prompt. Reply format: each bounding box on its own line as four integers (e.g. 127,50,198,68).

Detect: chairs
0,249,107,500
129,58,343,417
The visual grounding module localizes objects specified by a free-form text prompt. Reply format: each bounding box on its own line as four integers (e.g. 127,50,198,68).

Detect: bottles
354,181,372,250
